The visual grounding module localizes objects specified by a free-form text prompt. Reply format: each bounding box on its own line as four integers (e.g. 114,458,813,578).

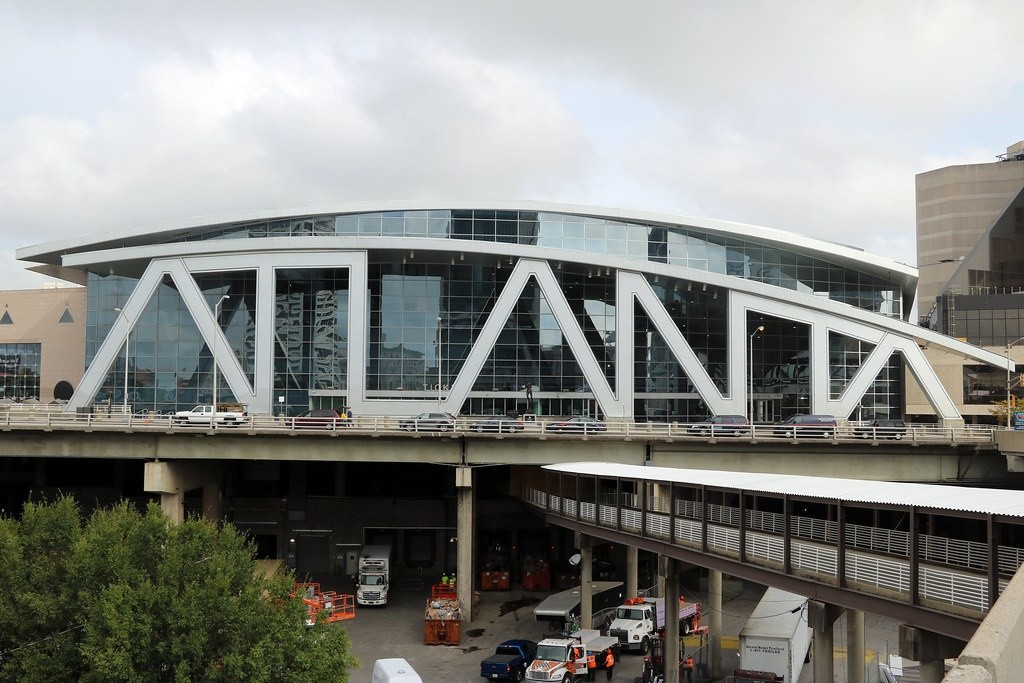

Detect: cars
545,415,607,435
469,416,525,434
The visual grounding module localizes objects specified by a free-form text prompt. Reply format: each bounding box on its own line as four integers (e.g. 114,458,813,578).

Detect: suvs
396,411,458,433
772,414,839,439
284,406,351,430
852,420,907,440
686,413,751,438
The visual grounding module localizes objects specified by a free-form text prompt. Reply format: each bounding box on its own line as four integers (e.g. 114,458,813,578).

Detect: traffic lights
1020,374,1024,387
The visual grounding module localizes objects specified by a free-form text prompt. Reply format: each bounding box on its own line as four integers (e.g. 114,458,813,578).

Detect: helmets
451,573,455,576
588,652,592,655
607,650,611,653
443,573,445,575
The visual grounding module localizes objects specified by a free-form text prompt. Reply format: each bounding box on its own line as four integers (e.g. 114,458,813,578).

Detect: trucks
732,586,813,683
351,543,394,609
523,627,622,683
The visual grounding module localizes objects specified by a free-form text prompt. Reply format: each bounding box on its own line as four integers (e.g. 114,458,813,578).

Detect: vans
371,658,424,683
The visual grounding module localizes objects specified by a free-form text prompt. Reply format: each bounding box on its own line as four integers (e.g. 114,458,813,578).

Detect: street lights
212,294,231,426
749,325,765,436
436,316,443,413
114,307,130,419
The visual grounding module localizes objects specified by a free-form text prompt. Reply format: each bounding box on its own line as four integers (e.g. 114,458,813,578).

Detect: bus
532,581,625,640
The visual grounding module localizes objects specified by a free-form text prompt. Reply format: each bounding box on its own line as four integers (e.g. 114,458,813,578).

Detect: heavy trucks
606,595,701,656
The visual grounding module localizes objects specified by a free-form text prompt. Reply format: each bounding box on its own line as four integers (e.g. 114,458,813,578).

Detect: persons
351,575,358,593
572,647,596,683
521,381,533,404
340,406,354,430
602,649,614,681
681,654,694,683
440,573,457,593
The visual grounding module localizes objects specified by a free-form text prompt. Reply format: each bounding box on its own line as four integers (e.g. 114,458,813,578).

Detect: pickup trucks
479,638,538,683
174,405,250,428
